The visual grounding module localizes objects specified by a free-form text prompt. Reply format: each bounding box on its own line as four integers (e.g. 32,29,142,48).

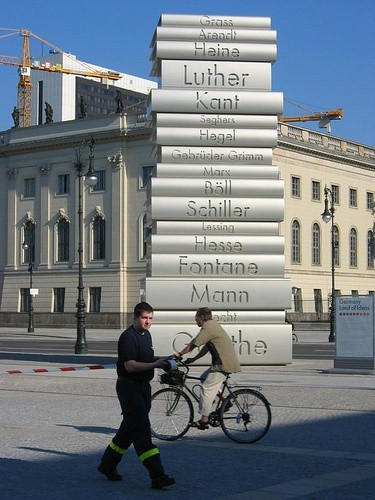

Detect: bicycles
148,355,272,444
289,321,298,343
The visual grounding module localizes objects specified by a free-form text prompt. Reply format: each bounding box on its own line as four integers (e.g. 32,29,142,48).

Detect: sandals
217,400,233,411
188,420,209,428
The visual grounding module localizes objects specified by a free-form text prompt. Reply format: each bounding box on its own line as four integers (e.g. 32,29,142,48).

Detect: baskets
160,370,185,386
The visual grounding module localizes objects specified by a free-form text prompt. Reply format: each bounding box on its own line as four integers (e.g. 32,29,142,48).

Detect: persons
96,300,178,490
170,307,242,429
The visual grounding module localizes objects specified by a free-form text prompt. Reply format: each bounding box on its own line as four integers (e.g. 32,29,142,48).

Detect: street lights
21,229,35,333
74,136,99,353
320,187,336,343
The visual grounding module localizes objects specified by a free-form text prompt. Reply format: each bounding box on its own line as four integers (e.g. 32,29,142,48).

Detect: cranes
1,28,122,128
278,97,344,133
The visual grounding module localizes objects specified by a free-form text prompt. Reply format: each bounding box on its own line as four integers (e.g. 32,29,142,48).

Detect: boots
139,447,176,489
97,441,127,481
